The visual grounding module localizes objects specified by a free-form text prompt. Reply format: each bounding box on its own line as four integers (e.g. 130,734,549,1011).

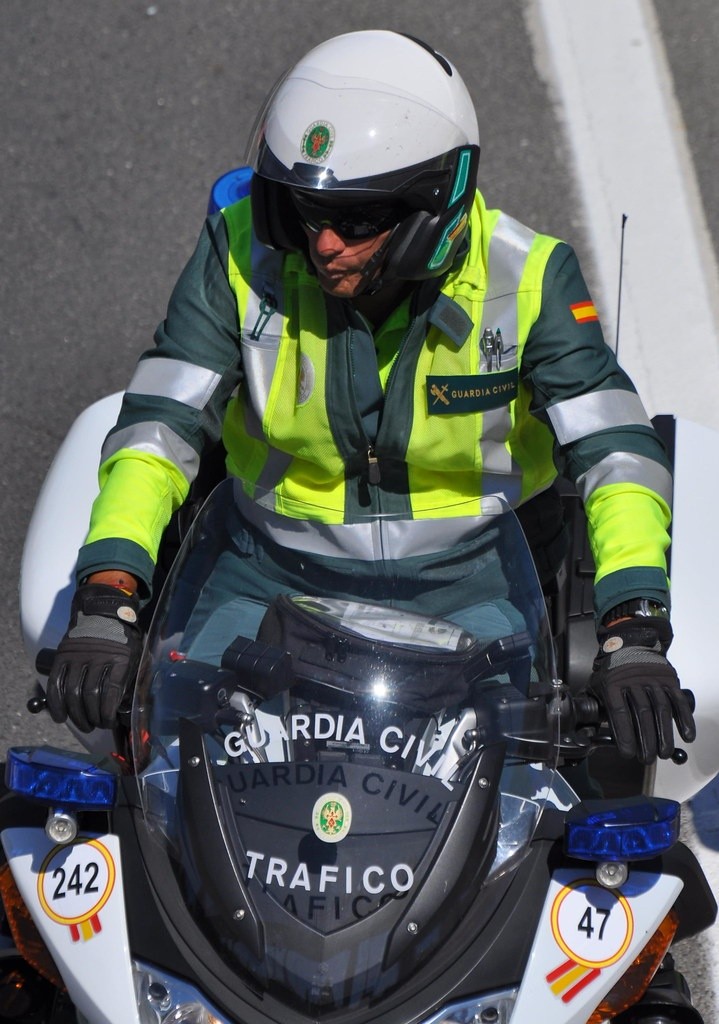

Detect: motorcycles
0,470,712,1024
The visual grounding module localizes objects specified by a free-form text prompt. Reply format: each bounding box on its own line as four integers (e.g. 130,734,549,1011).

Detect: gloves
576,607,692,762
51,577,154,754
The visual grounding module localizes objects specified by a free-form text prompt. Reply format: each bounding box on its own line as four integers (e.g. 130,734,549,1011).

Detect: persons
47,28,694,779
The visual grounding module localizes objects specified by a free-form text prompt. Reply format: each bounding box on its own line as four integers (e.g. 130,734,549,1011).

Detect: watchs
602,597,670,625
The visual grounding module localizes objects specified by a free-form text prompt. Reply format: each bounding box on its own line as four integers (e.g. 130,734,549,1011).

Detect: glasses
283,186,401,240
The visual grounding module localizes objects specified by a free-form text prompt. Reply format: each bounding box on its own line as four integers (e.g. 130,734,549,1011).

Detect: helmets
244,17,480,308
